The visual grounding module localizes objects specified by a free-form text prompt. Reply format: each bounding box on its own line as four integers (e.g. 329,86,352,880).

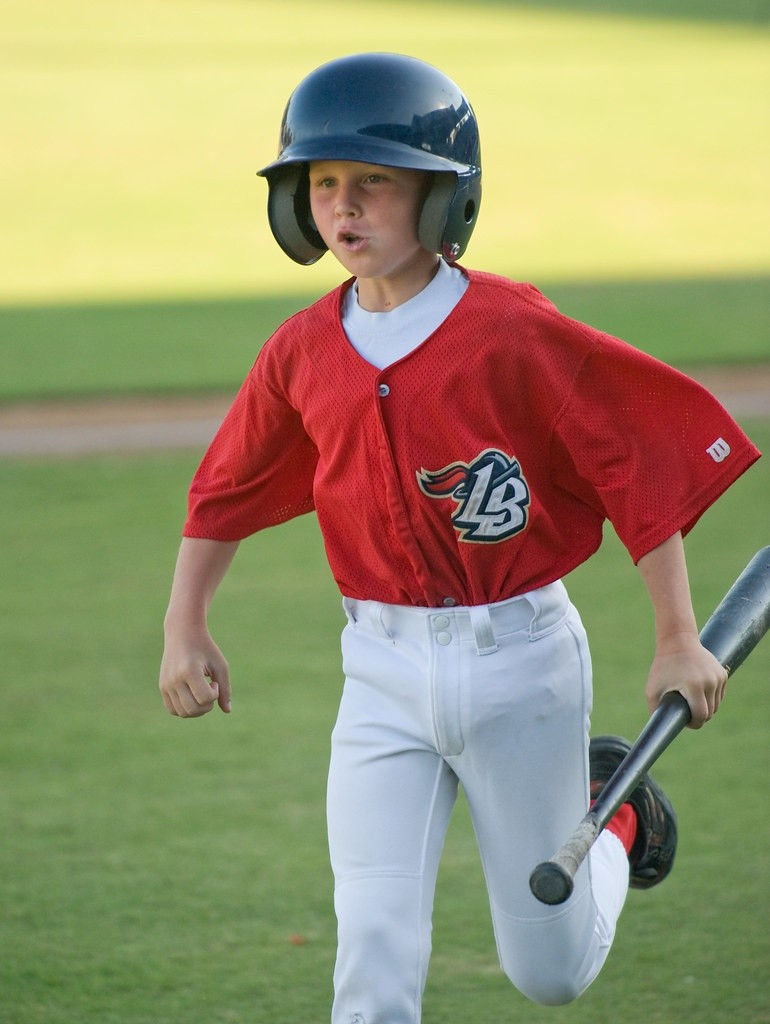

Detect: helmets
256,52,482,266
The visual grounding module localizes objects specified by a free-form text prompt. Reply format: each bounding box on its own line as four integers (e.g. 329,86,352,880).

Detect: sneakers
589,735,678,890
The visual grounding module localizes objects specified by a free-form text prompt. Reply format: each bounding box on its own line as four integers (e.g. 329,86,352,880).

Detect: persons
159,53,763,1024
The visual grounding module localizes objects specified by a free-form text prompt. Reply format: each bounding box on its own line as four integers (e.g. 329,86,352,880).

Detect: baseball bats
529,543,770,907
